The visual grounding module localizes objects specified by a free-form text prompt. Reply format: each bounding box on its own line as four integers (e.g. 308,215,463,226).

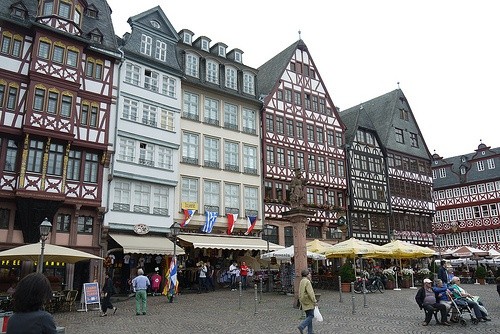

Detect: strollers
446,290,478,326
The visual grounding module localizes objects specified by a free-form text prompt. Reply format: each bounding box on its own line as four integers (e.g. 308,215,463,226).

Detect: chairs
43,289,79,313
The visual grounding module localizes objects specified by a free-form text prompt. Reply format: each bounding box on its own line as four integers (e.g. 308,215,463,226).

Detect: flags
243,216,258,235
202,211,219,233
161,257,179,296
179,210,196,228
226,213,239,235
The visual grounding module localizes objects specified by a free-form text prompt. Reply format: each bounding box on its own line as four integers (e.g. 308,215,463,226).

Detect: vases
402,279,411,288
383,280,396,290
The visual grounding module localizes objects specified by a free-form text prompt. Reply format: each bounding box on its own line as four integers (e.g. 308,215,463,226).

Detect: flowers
383,268,395,279
419,268,430,274
402,268,415,278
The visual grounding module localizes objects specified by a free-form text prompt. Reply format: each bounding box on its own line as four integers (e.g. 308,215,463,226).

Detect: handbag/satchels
314,306,323,322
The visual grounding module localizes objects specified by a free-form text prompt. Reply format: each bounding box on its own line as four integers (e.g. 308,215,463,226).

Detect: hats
137,268,145,275
452,276,459,282
300,270,309,277
423,278,432,283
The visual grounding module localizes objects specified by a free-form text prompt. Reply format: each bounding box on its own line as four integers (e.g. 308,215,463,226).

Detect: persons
197,260,215,293
239,261,248,290
415,260,492,326
131,268,150,315
100,271,117,317
297,269,319,334
6,272,58,334
229,259,238,292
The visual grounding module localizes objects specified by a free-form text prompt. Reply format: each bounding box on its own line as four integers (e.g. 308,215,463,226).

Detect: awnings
106,231,186,255
176,234,286,251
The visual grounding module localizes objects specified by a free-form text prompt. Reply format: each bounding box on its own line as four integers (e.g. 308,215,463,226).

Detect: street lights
334,226,343,243
171,221,181,256
263,223,273,292
38,217,53,271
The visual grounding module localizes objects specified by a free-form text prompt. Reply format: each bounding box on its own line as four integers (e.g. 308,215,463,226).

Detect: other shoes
483,317,491,320
112,308,117,314
478,318,485,322
231,289,235,292
136,312,140,315
422,321,428,326
143,312,146,315
100,313,107,317
297,327,303,334
235,288,237,291
204,291,208,293
152,294,155,297
441,322,451,326
197,291,201,294
449,317,458,323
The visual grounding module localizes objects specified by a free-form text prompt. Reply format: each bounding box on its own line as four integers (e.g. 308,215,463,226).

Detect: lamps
338,217,345,226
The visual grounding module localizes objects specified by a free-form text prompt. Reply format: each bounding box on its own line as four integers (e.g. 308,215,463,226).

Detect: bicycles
353,275,384,294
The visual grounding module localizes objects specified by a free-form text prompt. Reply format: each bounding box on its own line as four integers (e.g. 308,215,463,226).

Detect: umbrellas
306,239,500,291
0,241,106,274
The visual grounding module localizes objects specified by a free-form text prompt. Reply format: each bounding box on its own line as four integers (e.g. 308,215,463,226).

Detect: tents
259,245,327,274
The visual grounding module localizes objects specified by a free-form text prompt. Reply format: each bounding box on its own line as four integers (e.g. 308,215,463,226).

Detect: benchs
424,308,472,325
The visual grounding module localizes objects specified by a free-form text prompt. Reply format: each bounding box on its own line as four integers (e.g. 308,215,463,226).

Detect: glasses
457,279,461,282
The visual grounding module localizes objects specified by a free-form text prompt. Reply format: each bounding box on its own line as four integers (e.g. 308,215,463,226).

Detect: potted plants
475,266,486,285
336,257,355,292
